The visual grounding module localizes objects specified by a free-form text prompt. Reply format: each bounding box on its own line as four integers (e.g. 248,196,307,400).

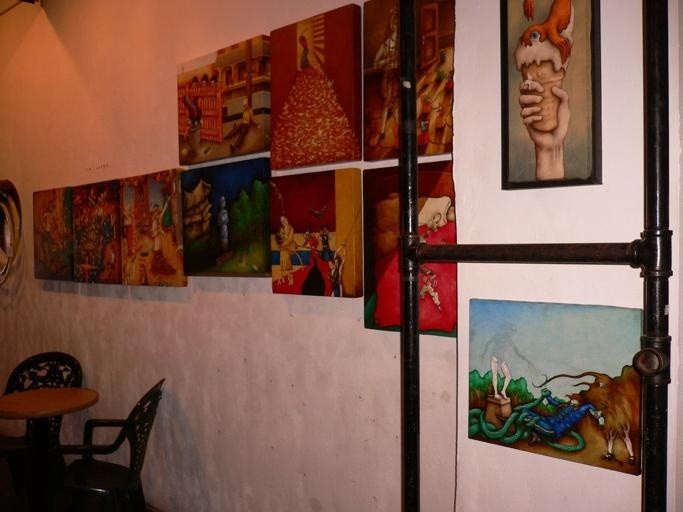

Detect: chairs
1,351,168,511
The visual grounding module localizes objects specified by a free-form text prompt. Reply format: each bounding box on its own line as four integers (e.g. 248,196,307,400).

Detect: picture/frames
498,1,604,192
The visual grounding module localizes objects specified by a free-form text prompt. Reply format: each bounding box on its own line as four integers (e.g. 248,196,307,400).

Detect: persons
519,80,570,180
480,322,536,401
275,213,346,298
225,98,263,152
